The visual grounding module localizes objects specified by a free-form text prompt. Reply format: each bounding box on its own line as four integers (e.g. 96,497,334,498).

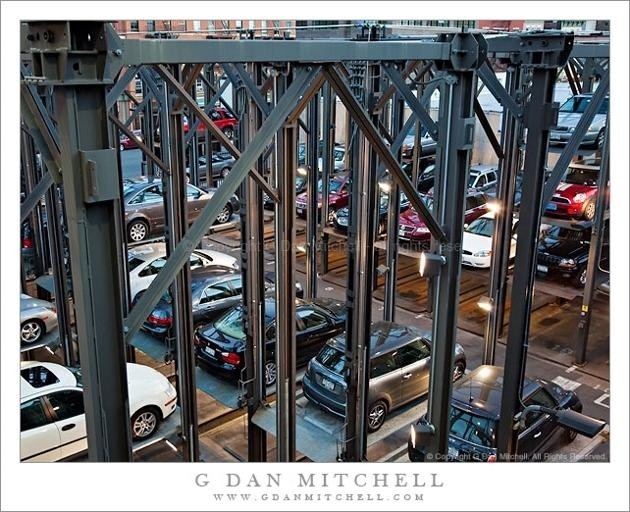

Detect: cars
20,292,180,463
122,179,242,243
128,248,240,310
120,129,143,151
301,319,468,434
549,92,610,151
132,269,305,344
192,294,346,389
406,364,584,463
